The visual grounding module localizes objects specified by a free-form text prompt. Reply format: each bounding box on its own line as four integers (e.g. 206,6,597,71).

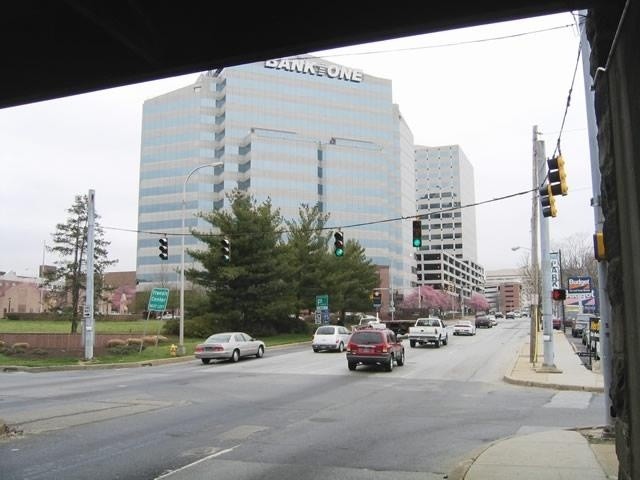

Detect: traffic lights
553,289,566,300
536,186,551,217
335,230,342,257
220,239,230,263
546,160,561,195
414,221,420,246
158,238,168,261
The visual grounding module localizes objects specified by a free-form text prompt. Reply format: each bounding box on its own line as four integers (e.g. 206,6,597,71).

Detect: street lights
40,238,51,314
388,252,414,320
452,273,464,313
178,161,224,347
8,297,13,313
511,244,532,252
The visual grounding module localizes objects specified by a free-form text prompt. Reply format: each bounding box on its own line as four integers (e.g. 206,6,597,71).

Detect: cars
542,314,562,331
312,308,529,372
289,315,304,321
195,329,266,364
109,310,120,315
157,314,178,320
563,304,600,363
